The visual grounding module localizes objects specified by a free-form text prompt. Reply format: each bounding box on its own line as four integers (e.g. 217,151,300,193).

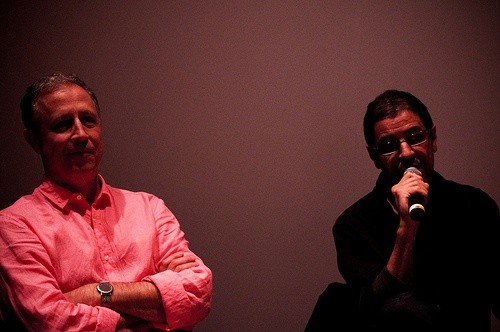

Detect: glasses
369,126,430,156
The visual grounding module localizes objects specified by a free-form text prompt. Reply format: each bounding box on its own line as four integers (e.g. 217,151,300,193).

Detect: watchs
96,281,116,304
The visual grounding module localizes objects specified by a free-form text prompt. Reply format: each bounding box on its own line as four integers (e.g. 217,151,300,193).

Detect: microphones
404,167,427,222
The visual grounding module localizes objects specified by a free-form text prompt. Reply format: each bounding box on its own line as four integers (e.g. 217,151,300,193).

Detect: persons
303,88,500,332
0,72,214,332
